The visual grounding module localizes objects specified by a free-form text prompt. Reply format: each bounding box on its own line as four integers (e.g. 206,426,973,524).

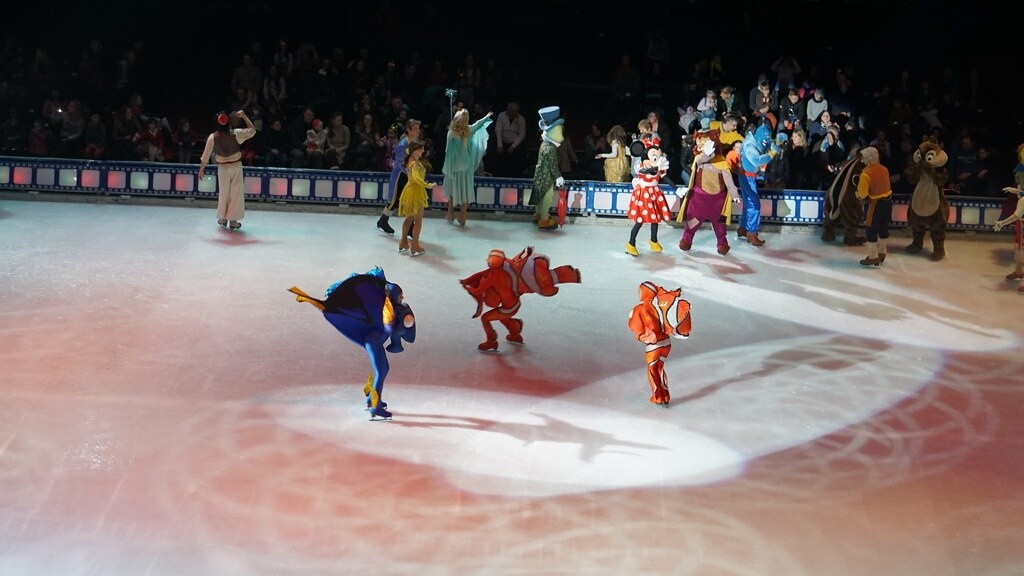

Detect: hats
697,117,711,133
538,106,564,131
218,114,228,126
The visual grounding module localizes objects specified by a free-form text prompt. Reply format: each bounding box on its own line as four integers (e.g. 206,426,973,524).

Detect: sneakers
532,213,558,230
457,216,467,227
230,220,241,232
369,408,392,421
446,213,455,224
1017,286,1024,295
376,214,395,236
365,398,388,411
860,253,887,269
626,241,639,257
737,227,766,247
1005,272,1024,282
218,219,228,226
649,239,662,252
408,222,414,240
506,333,525,346
410,241,425,257
650,396,670,409
478,341,500,353
398,239,409,254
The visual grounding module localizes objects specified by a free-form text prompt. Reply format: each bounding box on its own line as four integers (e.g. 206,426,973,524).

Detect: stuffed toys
529,106,565,229
823,144,882,246
678,128,742,255
627,140,674,255
906,135,950,262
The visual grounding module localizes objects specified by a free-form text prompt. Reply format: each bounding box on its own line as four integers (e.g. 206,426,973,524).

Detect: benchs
173,133,211,164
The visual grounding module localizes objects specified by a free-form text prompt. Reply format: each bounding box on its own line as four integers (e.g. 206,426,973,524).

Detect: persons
628,281,672,405
465,248,524,352
594,124,631,182
741,124,788,246
288,280,416,418
855,147,893,266
398,140,426,253
557,127,581,179
2,30,493,178
611,29,1007,195
494,101,525,179
992,170,1024,293
998,142,1024,225
584,120,606,180
441,108,493,224
376,119,421,237
198,110,257,230
